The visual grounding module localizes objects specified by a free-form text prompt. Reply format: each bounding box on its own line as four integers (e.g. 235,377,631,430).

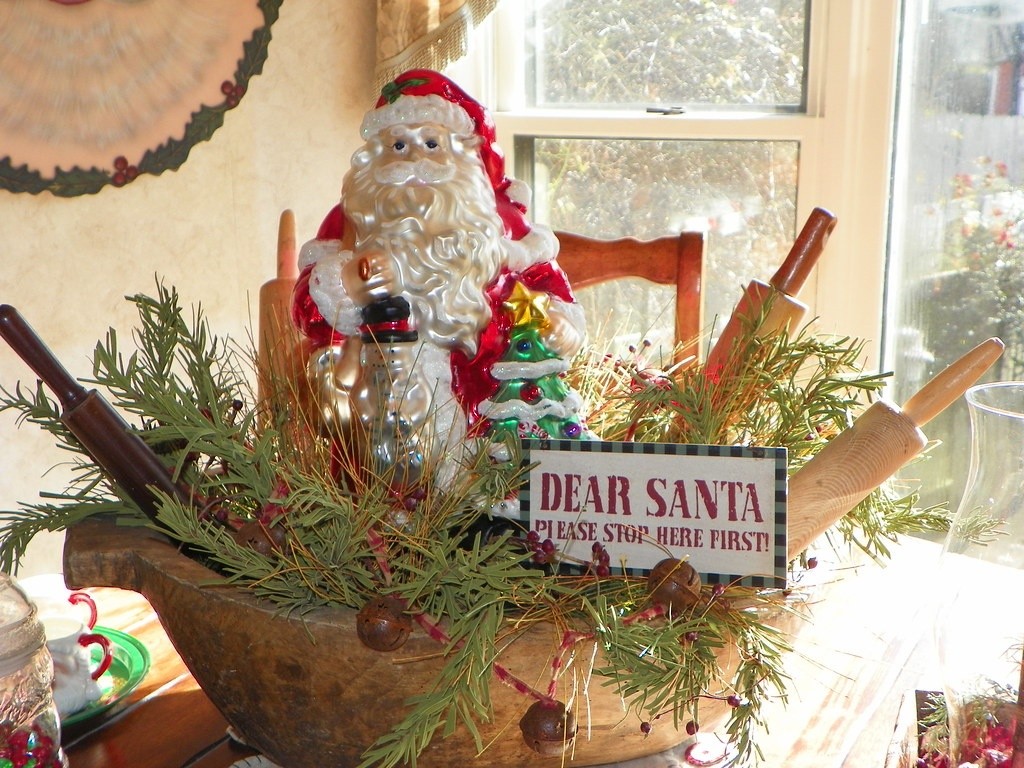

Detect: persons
287,69,602,551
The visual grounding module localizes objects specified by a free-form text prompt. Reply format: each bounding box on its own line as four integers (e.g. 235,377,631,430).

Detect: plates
60,625,151,727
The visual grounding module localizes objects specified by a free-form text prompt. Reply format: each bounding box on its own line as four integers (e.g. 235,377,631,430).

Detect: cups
18,572,112,715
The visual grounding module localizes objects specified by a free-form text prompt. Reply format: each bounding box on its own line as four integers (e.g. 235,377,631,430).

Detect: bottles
930,379,1023,767
0,572,70,768
